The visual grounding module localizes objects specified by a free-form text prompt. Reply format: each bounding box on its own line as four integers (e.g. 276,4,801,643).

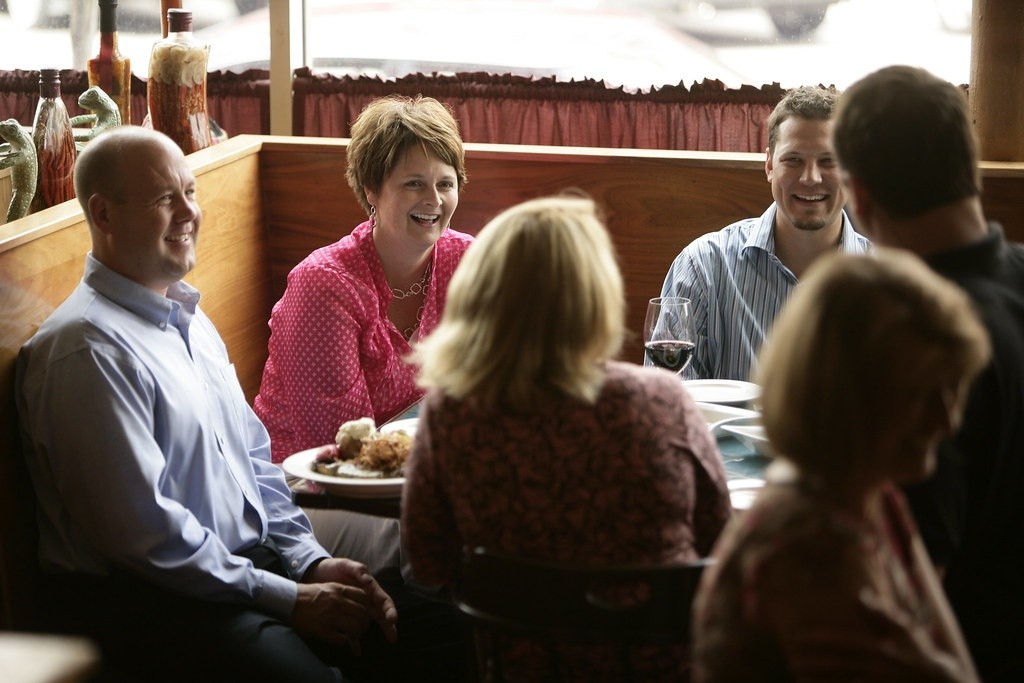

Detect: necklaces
391,265,433,339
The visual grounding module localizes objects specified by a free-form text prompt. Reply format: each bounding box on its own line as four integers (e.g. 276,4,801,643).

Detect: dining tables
294,385,774,524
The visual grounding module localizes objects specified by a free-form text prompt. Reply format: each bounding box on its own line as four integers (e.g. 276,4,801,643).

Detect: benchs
0,135,1024,683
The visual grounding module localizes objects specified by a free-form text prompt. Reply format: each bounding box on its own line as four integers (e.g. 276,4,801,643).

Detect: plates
692,403,761,442
727,478,767,510
681,378,763,406
379,418,419,440
283,444,406,498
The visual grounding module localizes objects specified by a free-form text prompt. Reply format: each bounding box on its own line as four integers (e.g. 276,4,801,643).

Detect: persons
651,88,869,381
254,92,474,463
0,126,403,683
400,186,730,683
833,65,1024,683
690,241,994,682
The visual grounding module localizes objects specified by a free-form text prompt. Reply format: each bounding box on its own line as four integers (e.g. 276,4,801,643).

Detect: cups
643,297,697,375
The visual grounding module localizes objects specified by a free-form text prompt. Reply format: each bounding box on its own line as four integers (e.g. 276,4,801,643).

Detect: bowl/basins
720,425,774,460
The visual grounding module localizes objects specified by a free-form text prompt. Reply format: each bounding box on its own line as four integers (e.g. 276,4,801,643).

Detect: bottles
87,0,132,128
30,69,76,207
147,7,212,156
159,0,183,39
